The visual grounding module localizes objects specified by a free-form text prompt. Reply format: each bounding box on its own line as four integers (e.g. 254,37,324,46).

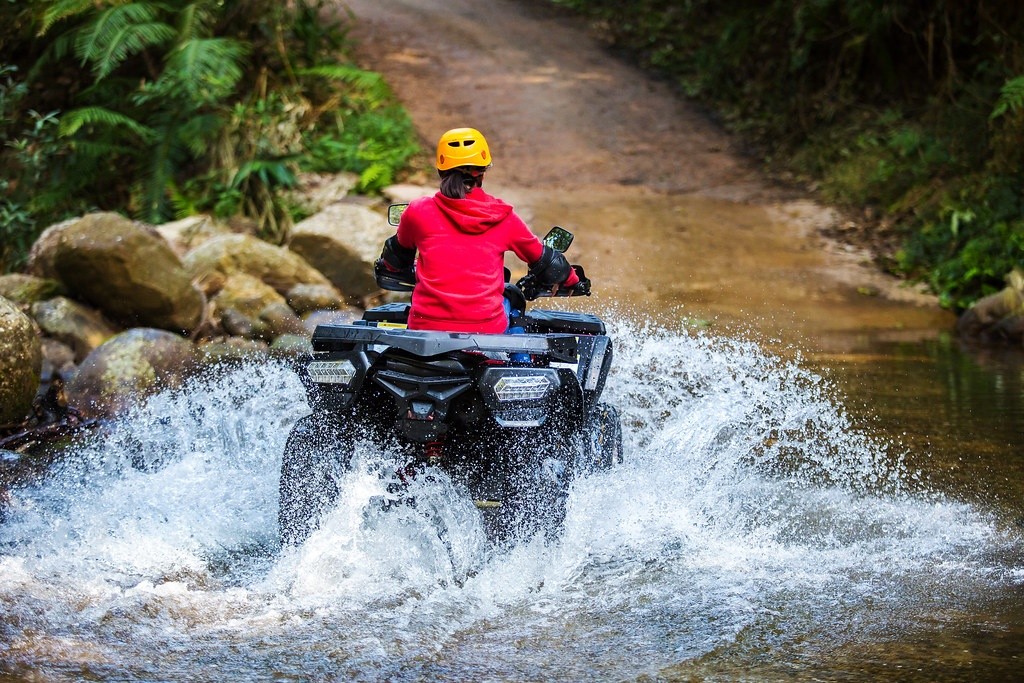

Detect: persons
380,127,580,367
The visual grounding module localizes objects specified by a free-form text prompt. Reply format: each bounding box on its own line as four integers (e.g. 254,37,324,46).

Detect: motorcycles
278,201,623,558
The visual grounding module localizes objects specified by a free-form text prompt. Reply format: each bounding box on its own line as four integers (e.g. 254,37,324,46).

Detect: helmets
434,127,493,172
